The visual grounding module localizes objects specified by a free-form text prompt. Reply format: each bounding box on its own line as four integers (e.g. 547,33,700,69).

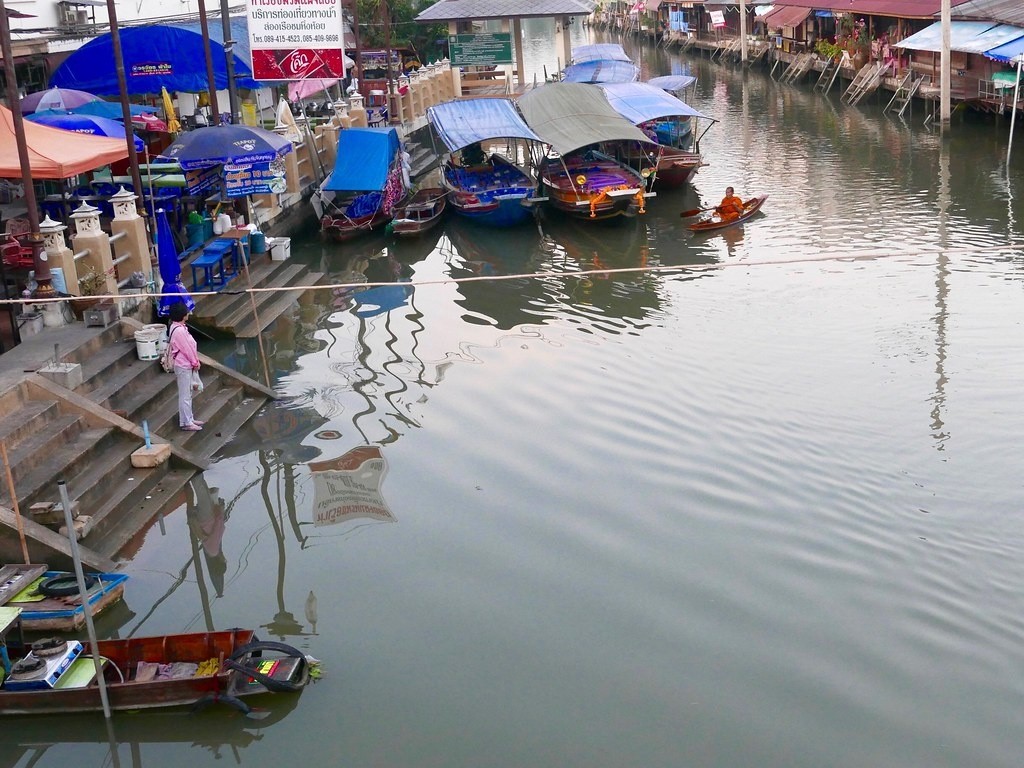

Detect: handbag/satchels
190,369,203,399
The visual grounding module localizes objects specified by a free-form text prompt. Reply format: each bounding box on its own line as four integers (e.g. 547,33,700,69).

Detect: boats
561,43,720,191
0,606,319,715
687,194,770,232
514,81,666,223
0,563,131,634
312,127,415,241
425,97,550,228
384,187,448,238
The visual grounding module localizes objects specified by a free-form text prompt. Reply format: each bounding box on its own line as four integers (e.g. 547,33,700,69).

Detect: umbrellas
19,84,106,117
29,111,145,153
123,111,168,132
155,208,197,317
24,107,73,122
151,120,293,170
69,98,161,122
162,87,183,136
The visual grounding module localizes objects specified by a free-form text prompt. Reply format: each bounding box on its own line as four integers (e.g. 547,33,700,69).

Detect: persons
712,186,744,223
192,475,228,575
168,302,205,431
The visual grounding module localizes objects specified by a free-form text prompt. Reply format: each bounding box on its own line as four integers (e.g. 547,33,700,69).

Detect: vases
853,51,868,69
82,301,119,327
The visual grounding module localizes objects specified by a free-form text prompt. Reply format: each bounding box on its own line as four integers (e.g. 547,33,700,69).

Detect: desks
90,176,161,187
993,71,1024,97
128,163,182,175
191,230,251,291
156,175,189,187
368,116,386,127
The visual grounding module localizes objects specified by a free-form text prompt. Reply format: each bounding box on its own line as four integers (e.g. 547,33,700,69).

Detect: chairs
0,218,34,267
42,182,184,232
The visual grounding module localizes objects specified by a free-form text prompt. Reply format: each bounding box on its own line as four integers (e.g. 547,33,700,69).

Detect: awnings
891,20,998,52
950,24,1024,55
983,36,1024,68
753,4,812,29
645,0,663,12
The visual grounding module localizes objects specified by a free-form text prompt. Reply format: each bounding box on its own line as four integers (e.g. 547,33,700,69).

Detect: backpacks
160,325,183,373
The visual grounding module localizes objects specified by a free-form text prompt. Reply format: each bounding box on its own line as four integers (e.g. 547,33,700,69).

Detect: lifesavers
38,572,94,596
223,639,310,692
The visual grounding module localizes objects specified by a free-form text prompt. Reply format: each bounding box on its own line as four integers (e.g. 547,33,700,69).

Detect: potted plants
814,38,845,63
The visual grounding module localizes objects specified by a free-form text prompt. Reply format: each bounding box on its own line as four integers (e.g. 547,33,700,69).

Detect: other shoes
181,423,203,431
193,420,206,424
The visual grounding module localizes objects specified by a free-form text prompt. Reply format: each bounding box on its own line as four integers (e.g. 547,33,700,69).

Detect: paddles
679,204,733,217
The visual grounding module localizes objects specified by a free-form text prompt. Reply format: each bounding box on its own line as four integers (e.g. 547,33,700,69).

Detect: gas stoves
3,637,84,692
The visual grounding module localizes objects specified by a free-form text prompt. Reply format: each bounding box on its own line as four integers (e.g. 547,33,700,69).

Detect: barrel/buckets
271,236,291,260
235,245,250,265
251,234,265,253
186,221,213,244
213,215,231,234
134,323,168,361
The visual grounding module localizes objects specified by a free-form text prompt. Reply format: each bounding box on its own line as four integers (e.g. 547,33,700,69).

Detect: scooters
287,97,337,117
181,106,207,129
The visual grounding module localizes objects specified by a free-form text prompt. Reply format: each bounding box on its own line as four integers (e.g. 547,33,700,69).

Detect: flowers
833,16,870,56
81,261,116,296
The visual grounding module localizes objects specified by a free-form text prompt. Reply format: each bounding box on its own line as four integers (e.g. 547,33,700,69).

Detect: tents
0,104,158,259
60,14,290,131
